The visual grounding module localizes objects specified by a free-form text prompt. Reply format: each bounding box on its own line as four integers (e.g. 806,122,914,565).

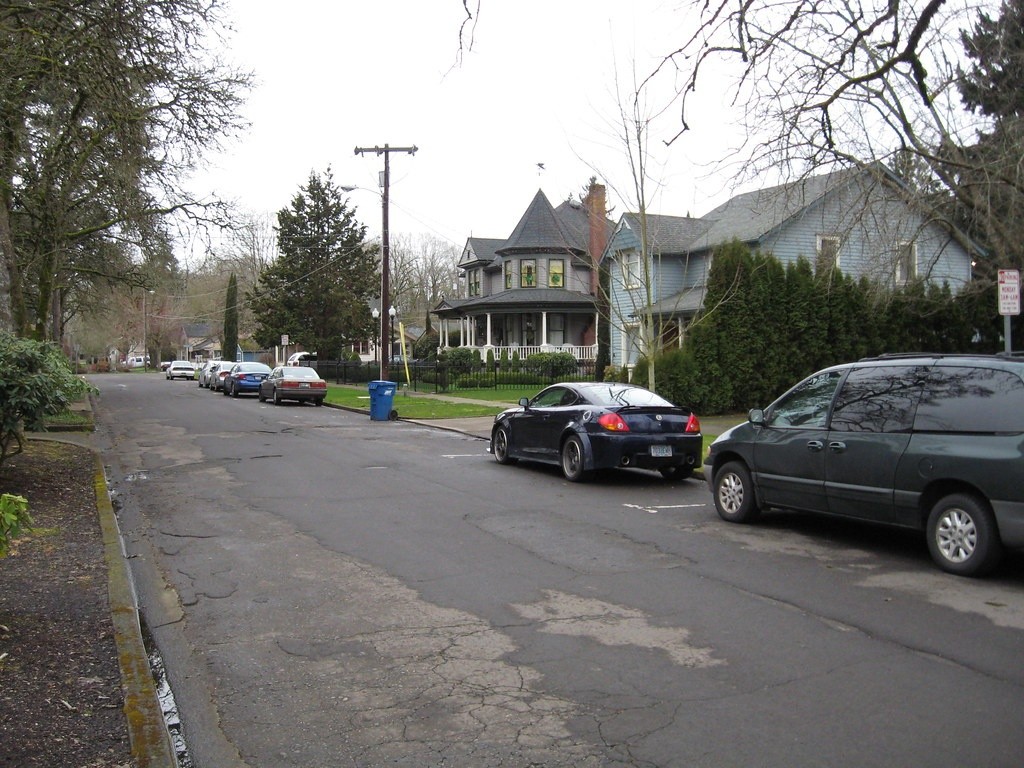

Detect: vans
702,354,1024,575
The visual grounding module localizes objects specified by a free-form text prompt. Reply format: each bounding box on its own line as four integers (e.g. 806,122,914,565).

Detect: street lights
338,184,388,380
370,307,379,367
389,305,396,367
143,286,155,373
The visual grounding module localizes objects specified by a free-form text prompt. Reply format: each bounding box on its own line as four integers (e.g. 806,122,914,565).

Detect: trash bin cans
368,380,398,421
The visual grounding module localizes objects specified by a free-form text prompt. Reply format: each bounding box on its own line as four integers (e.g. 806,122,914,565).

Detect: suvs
288,352,318,371
123,357,150,368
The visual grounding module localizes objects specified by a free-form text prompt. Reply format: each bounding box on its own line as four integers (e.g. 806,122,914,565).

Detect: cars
198,361,220,388
489,379,704,483
210,362,237,391
156,362,170,371
258,366,327,406
166,361,195,380
223,362,273,397
390,355,412,362
